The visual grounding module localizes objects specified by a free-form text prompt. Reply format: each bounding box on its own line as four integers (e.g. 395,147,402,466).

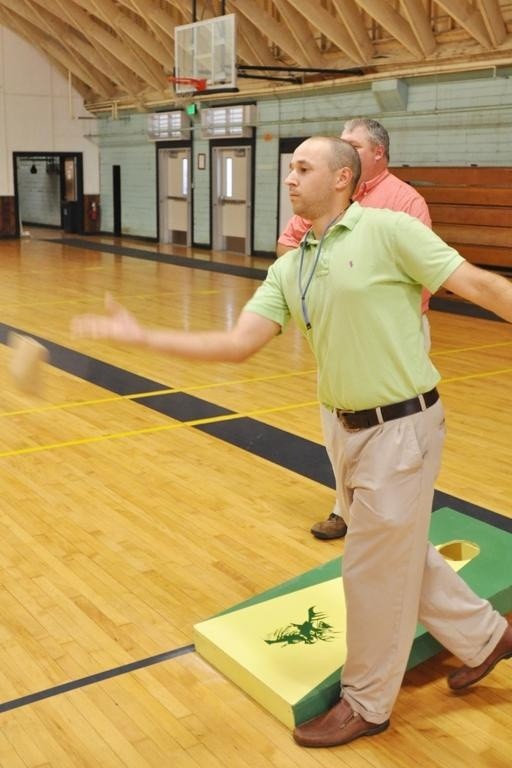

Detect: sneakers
310,514,346,539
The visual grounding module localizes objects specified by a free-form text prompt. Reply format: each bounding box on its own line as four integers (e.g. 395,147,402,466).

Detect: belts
335,387,438,434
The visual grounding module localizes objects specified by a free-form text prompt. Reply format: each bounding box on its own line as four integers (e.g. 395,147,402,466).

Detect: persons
73,134,512,748
276,117,435,540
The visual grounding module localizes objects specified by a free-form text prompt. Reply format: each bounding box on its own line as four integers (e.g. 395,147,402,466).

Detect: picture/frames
197,154,205,170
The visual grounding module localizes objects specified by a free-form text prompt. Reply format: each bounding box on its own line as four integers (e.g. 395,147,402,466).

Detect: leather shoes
446,624,512,688
294,698,390,748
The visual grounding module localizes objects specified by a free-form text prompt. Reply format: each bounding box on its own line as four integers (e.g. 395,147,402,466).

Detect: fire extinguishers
91,203,97,220
